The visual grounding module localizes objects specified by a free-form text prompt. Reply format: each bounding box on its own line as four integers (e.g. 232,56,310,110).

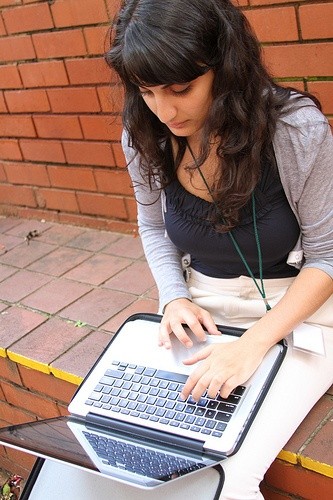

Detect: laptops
0,312,289,491
67,420,219,487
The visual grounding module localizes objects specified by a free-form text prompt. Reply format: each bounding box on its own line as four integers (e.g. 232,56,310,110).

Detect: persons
106,1,332,404
